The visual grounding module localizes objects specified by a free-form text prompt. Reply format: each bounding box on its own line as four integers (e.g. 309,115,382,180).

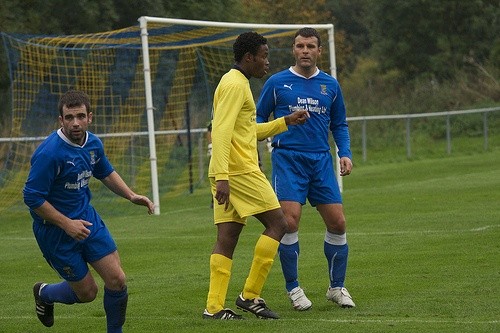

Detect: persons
256,27,356,311
23,90,155,333
207,112,264,208
202,32,310,319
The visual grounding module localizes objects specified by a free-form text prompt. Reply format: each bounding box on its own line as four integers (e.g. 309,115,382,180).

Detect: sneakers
202,308,246,321
236,292,280,320
33,283,54,327
326,285,355,307
287,286,312,312
